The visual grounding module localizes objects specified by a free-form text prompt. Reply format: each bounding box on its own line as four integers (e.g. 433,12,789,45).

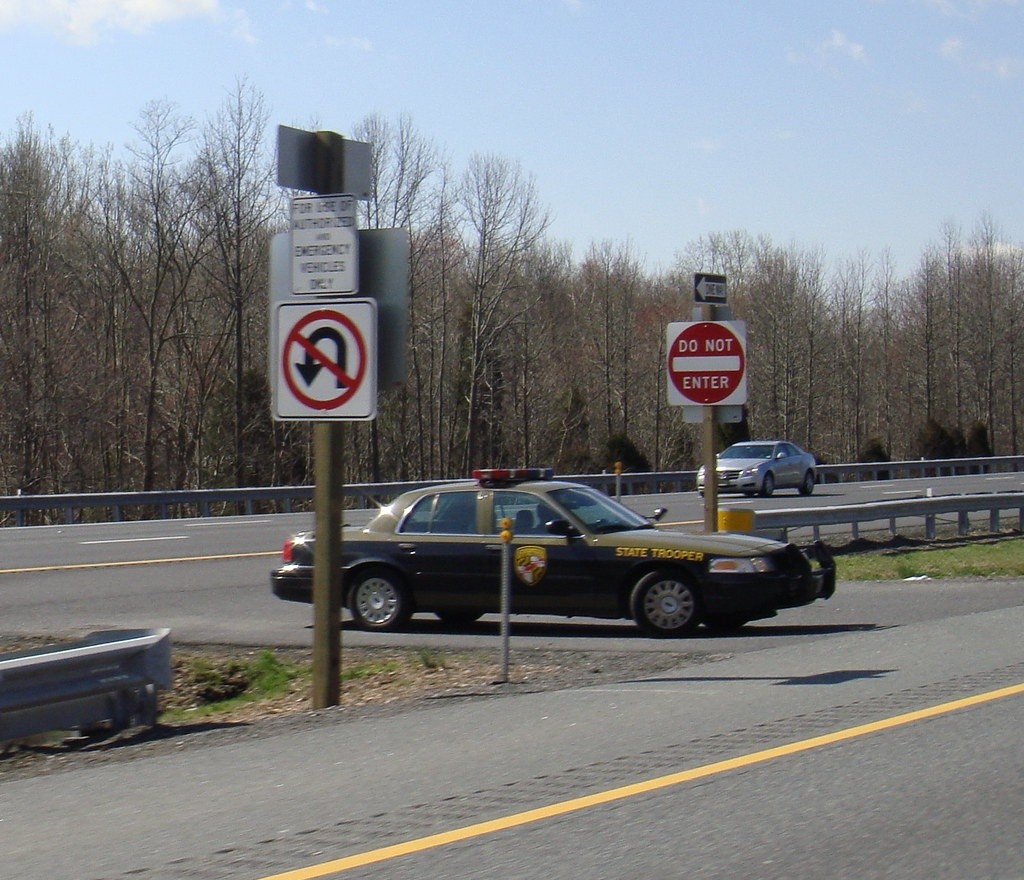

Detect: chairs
511,508,538,535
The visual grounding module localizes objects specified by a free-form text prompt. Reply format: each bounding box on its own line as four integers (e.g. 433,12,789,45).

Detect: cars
266,467,838,640
694,440,819,497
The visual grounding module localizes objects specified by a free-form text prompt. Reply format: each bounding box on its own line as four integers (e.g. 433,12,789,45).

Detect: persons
515,505,554,535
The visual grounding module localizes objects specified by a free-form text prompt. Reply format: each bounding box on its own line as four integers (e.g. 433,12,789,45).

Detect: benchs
409,520,470,534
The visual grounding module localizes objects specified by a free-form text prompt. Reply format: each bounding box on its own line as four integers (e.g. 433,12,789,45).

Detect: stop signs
665,322,746,406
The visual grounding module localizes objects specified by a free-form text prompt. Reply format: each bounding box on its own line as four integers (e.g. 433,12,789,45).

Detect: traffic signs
692,272,728,305
